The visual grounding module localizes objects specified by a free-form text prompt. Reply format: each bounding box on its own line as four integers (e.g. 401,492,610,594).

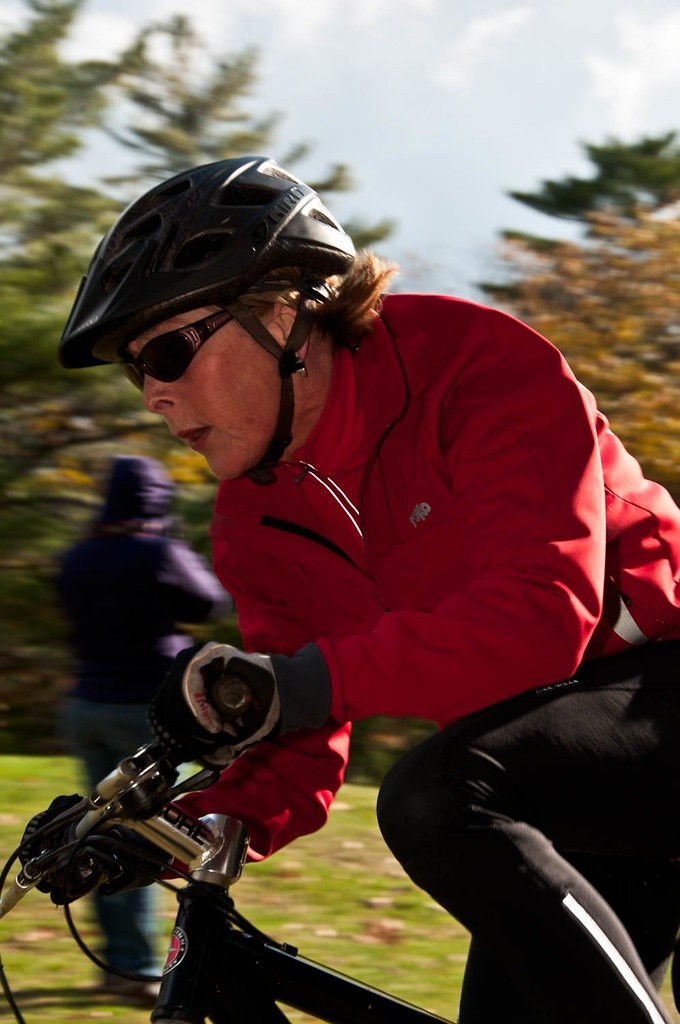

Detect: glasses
119,310,233,391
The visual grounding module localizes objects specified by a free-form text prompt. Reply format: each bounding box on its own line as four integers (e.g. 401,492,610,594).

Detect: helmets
56,156,357,369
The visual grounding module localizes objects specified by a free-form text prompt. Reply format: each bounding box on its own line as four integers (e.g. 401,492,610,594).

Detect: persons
18,156,680,1024
43,453,232,1003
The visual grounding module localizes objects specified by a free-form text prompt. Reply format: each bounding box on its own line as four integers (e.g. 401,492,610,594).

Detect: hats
102,456,175,515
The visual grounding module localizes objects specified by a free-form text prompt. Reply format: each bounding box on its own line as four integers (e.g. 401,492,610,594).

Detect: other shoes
106,972,162,1004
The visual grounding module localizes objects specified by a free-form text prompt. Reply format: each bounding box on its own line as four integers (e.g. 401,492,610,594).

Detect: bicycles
0,675,680,1023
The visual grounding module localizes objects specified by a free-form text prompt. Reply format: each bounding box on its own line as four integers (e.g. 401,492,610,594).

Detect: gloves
146,640,281,770
18,793,156,905
